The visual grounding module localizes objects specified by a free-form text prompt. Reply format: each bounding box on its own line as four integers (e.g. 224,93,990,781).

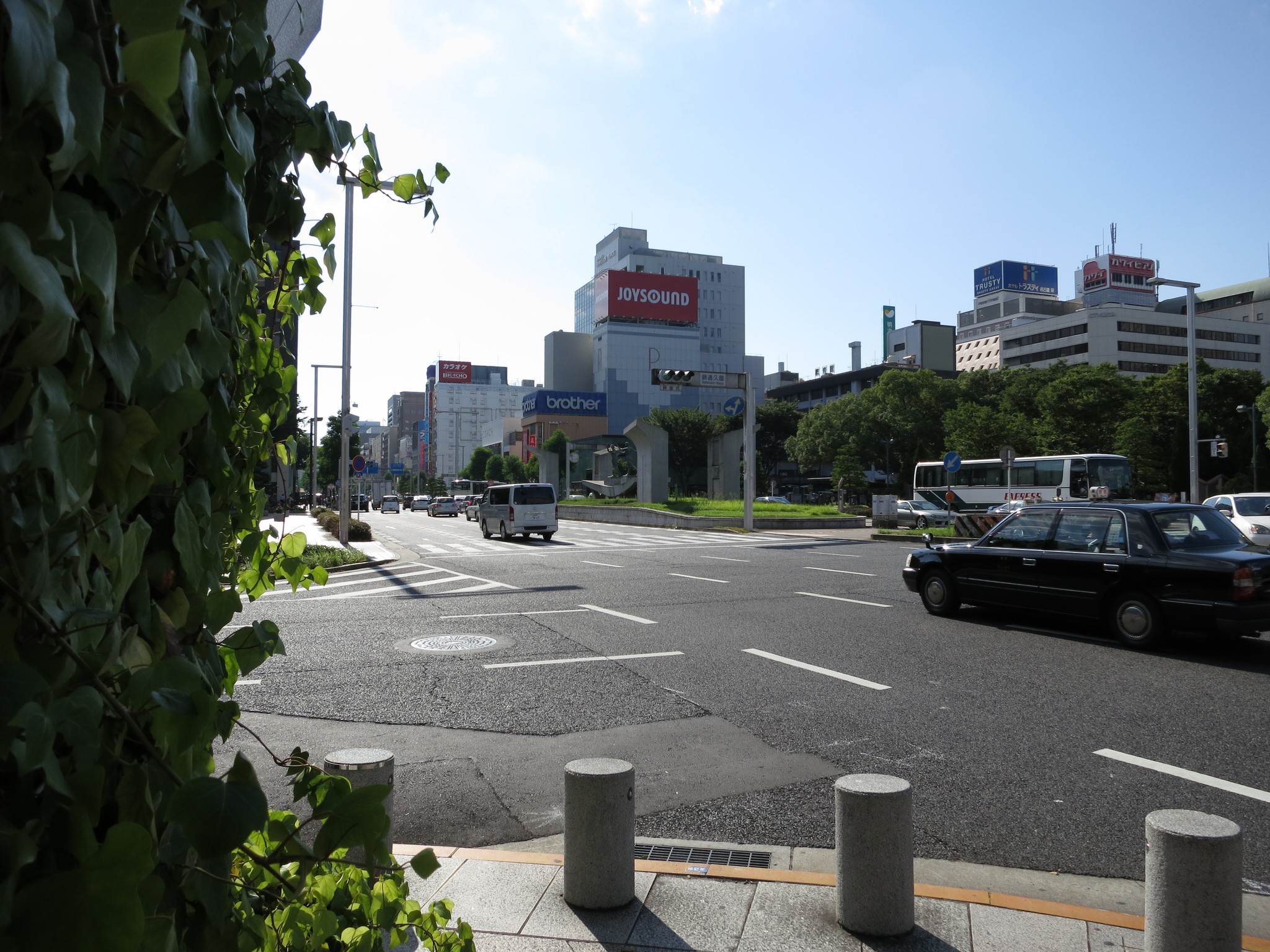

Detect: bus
914,453,1136,513
807,477,880,507
450,479,507,495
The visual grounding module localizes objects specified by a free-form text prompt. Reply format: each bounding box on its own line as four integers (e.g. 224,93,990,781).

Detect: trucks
370,480,392,510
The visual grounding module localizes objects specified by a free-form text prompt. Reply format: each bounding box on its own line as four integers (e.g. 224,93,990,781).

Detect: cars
986,498,1052,514
380,495,400,513
1191,493,1270,548
453,495,488,522
566,493,593,499
294,493,371,512
904,486,1270,649
403,495,433,512
756,497,791,504
897,500,958,529
397,493,403,501
427,496,458,517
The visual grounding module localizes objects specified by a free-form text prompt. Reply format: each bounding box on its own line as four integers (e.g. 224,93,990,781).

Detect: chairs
961,478,971,485
973,478,980,485
979,478,985,484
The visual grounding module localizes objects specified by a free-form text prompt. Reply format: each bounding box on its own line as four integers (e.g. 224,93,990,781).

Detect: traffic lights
1217,443,1228,458
658,369,694,383
344,414,360,436
364,460,374,468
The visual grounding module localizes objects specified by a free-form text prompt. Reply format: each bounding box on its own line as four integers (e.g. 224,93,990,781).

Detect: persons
280,494,285,504
288,494,295,501
1168,493,1181,503
1079,482,1090,497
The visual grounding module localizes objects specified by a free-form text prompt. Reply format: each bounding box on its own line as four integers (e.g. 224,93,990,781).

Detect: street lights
432,455,444,476
1147,277,1200,504
889,439,905,497
309,417,323,511
1236,405,1258,492
403,430,437,496
435,410,480,480
451,445,464,472
334,174,434,543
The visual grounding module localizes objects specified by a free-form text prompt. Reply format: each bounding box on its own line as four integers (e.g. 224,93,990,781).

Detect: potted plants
283,505,290,517
274,508,285,521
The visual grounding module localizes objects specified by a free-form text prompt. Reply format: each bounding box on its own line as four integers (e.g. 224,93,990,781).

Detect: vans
476,484,558,541
